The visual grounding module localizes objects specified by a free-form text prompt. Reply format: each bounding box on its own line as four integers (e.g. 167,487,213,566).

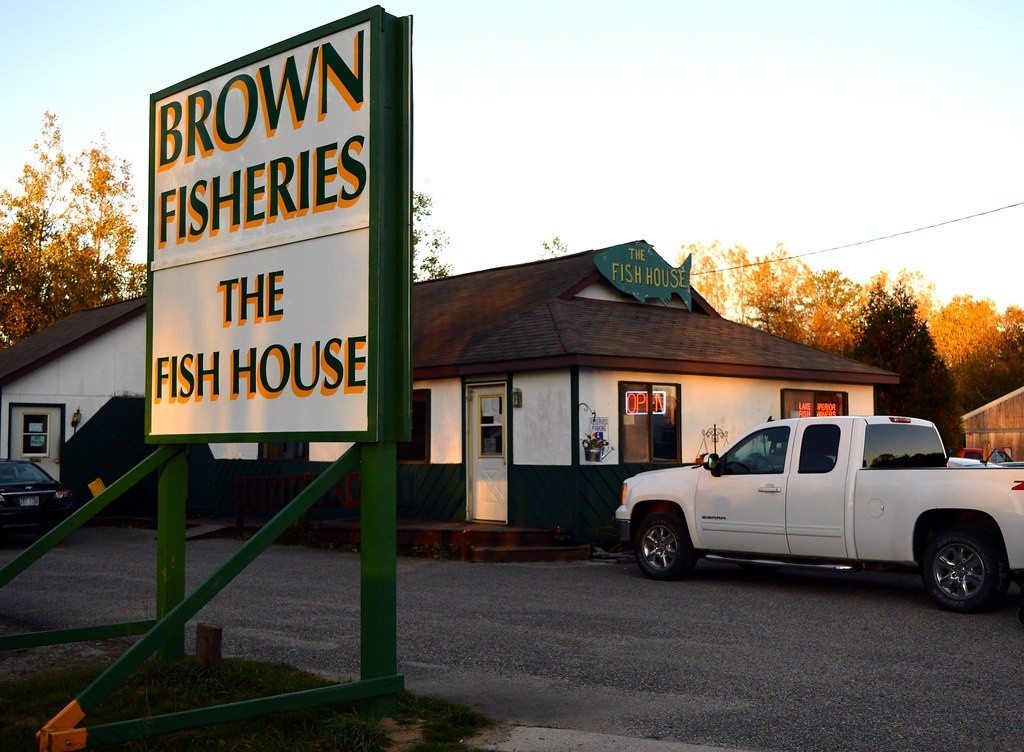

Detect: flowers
581,431,609,448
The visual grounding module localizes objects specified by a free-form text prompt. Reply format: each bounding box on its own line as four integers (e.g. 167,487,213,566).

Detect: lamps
72,408,82,428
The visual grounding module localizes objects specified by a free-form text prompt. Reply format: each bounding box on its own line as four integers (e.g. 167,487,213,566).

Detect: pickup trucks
615,415,1024,612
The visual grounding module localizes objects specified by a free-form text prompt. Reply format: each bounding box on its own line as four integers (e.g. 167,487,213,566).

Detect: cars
947,448,1024,469
0,457,74,535
